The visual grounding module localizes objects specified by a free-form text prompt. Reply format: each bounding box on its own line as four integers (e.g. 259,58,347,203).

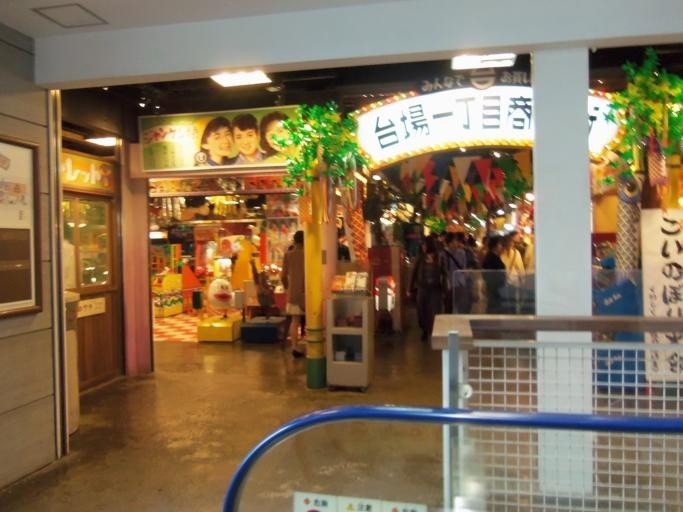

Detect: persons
400,229,535,346
255,109,304,164
589,237,617,287
282,243,306,338
276,229,307,359
335,227,352,261
229,112,266,167
188,116,236,169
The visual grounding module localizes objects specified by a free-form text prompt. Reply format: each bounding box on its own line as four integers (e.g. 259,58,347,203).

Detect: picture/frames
1,135,44,318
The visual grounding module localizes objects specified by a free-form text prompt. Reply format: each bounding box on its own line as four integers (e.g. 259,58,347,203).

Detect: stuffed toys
208,278,234,311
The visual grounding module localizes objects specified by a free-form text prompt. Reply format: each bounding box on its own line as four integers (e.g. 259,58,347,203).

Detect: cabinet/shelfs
323,296,373,390
